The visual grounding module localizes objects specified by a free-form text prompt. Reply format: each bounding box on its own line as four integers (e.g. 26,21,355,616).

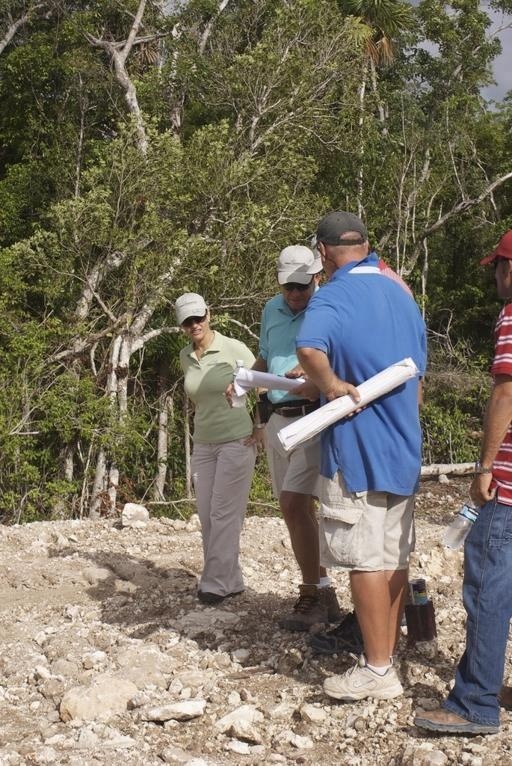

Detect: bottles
230,359,247,408
475,461,480,471
441,495,481,552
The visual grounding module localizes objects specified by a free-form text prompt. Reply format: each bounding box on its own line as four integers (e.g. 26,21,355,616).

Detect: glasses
182,316,206,326
283,276,314,290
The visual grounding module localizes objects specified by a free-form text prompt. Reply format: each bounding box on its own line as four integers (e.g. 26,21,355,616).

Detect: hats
175,293,207,325
277,210,368,285
480,230,512,264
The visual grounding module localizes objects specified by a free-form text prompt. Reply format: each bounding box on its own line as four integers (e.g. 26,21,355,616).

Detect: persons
413,231,511,735
173,293,266,602
223,246,344,632
283,254,413,655
295,210,426,701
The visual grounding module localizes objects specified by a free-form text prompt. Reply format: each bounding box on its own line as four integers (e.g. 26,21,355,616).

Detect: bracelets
254,424,265,429
474,461,491,474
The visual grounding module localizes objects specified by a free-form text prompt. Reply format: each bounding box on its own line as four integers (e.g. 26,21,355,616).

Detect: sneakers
198,590,246,605
310,612,363,652
323,652,404,700
414,707,499,734
284,584,341,629
